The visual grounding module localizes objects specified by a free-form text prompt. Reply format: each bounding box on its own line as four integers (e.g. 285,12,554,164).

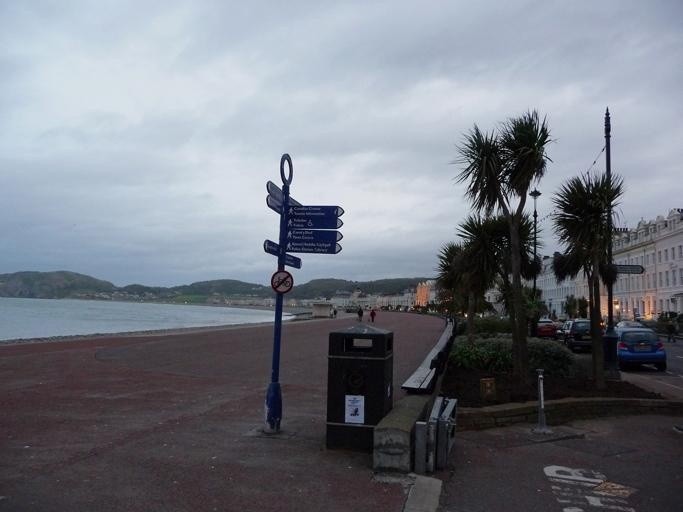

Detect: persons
333,309,337,318
357,306,363,322
663,320,676,344
369,309,375,323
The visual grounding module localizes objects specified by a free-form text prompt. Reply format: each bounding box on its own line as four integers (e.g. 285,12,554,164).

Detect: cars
614,321,667,370
536,318,592,352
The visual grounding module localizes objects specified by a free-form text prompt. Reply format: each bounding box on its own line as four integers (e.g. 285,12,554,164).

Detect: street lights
529,189,542,337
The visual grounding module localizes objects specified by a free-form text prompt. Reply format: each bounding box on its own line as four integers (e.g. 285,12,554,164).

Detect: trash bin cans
326,323,394,454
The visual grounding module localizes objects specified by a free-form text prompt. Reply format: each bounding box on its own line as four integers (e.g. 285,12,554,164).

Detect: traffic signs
263,180,344,269
